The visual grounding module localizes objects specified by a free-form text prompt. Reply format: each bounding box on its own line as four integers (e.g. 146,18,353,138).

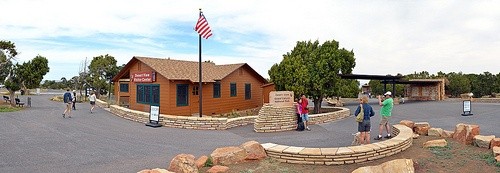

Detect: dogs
351,128,371,146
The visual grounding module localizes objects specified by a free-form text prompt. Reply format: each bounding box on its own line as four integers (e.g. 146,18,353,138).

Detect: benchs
3,95,24,107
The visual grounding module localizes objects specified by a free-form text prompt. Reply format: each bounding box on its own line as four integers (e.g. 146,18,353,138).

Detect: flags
194,12,212,39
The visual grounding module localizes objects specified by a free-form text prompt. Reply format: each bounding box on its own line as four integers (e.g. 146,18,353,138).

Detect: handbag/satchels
356,104,364,123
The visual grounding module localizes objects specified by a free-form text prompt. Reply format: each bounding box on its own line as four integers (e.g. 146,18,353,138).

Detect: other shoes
90,109,93,113
306,128,311,131
374,135,382,140
62,113,65,119
69,116,71,119
384,134,392,139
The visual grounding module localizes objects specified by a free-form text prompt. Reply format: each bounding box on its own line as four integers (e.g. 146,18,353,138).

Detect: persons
297,93,310,130
62,89,73,118
374,91,394,139
400,90,405,104
368,91,372,98
72,90,77,110
355,95,375,145
89,91,96,113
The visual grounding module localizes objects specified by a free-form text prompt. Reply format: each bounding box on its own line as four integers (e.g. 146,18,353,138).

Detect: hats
384,91,391,96
